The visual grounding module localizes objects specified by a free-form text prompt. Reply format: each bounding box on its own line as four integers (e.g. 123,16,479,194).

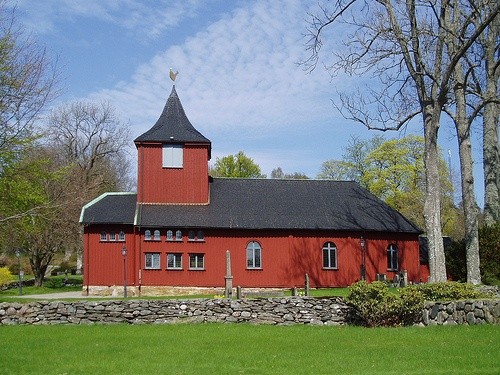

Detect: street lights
15,247,23,295
360,235,365,282
120,245,129,298
